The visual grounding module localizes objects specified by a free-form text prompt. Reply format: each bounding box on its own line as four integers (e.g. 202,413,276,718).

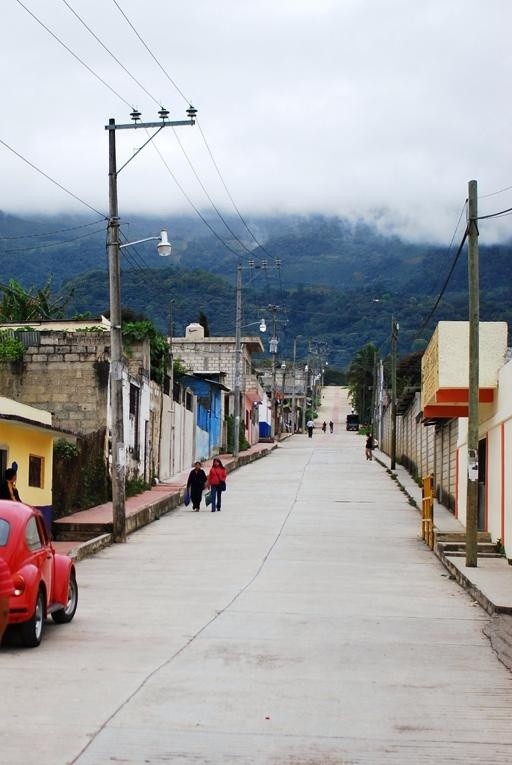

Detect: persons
329,420,334,433
323,421,327,432
207,456,226,512
0,467,22,503
187,461,207,513
365,432,373,461
306,417,314,438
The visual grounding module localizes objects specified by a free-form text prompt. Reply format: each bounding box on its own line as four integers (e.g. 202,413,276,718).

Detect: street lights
291,334,303,434
371,299,396,470
270,361,286,438
234,319,268,456
108,228,173,543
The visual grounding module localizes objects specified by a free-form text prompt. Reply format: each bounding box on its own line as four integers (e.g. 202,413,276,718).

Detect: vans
346,414,360,432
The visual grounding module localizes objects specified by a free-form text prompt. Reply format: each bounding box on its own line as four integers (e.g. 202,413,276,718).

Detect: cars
0,499,78,647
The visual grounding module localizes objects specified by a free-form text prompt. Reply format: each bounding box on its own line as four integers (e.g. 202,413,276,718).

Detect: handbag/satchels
184,492,190,506
205,491,212,506
219,480,226,491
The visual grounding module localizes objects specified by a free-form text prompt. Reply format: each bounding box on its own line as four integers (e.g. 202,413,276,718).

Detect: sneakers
190,506,221,512
